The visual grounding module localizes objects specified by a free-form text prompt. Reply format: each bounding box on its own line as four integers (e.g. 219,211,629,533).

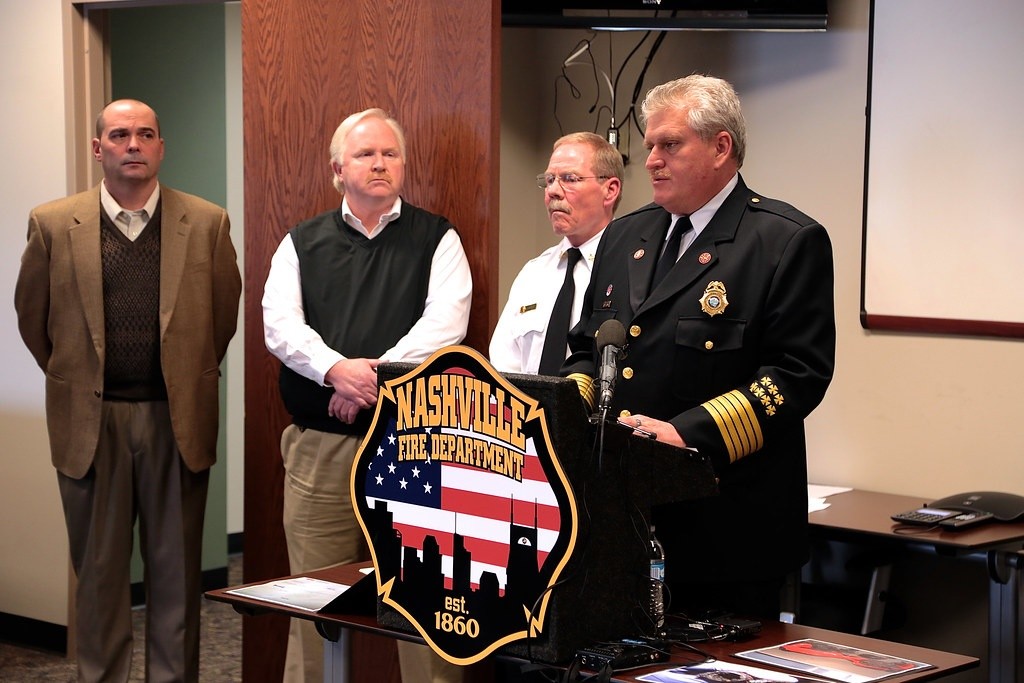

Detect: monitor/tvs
501,0,829,31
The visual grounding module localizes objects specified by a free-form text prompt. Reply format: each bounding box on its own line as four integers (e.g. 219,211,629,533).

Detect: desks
205,485,1024,683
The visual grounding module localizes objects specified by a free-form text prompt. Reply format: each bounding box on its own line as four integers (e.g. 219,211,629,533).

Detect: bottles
647,524,665,629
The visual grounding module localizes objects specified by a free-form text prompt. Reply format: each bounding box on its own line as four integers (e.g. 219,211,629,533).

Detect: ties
647,216,693,298
538,248,583,376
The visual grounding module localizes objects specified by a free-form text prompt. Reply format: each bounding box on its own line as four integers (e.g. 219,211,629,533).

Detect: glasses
536,173,610,189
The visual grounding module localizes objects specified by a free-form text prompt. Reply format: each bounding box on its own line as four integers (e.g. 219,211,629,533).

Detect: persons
488,132,625,375
561,76,836,623
15,98,242,682
263,109,470,682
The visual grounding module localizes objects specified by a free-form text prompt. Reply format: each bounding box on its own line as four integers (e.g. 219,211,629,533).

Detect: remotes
939,513,993,530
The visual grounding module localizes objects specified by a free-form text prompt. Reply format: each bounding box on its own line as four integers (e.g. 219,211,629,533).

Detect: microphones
597,319,626,408
